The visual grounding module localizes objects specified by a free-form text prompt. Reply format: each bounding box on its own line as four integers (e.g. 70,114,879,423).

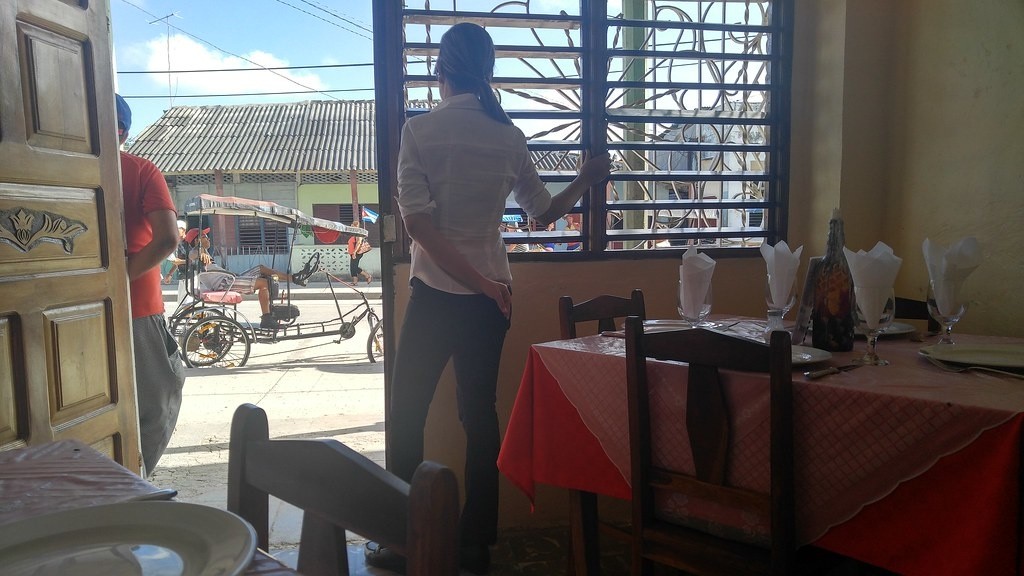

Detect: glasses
202,235,208,238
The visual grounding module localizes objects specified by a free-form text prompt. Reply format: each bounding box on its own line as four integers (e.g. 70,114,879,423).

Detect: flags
362,208,379,224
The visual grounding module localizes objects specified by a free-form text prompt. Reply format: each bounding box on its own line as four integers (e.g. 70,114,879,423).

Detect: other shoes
462,545,491,572
260,316,288,330
363,540,404,568
292,263,310,285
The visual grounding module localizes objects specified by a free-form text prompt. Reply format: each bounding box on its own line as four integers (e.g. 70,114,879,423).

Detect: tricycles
168,194,384,366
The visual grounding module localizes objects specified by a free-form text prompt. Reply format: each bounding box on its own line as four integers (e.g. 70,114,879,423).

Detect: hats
115,93,132,137
176,220,187,230
186,227,209,244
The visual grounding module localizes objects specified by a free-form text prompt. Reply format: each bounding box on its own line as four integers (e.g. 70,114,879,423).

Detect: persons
164,221,193,325
364,22,612,574
348,221,372,286
541,215,580,250
185,228,310,329
115,93,187,480
506,221,529,252
650,222,671,249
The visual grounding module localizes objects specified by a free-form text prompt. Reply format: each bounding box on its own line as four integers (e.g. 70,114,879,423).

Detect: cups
677,280,712,330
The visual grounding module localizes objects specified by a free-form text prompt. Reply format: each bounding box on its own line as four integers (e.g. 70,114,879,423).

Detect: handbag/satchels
354,236,372,254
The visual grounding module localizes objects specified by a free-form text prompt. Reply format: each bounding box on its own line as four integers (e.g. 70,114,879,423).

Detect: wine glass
927,278,968,344
764,273,797,336
851,286,895,366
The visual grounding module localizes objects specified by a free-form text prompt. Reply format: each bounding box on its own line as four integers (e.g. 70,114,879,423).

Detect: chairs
626,314,842,576
266,274,299,317
228,404,462,576
886,295,941,333
557,288,650,575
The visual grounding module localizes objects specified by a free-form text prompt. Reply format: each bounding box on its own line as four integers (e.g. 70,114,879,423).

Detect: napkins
922,236,984,322
761,240,804,311
678,243,717,316
842,240,902,332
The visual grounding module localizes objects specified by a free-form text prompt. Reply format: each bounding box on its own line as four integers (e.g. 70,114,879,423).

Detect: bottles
764,310,786,344
813,219,854,352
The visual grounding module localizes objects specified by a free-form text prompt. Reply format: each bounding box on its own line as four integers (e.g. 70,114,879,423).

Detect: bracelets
167,274,172,277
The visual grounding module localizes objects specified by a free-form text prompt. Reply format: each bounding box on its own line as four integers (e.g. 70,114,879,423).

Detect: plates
621,320,723,334
0,500,258,576
791,344,833,363
919,343,1024,367
855,321,915,334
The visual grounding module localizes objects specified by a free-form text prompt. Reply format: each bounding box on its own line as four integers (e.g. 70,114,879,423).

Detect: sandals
349,282,358,286
366,274,373,285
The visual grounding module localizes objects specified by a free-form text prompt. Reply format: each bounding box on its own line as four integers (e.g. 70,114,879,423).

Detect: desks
0,439,306,576
497,328,1024,576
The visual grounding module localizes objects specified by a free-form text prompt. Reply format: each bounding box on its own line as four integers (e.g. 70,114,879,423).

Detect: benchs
186,265,242,304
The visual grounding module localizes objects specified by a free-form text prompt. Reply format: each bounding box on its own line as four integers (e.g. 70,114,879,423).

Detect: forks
926,356,1024,379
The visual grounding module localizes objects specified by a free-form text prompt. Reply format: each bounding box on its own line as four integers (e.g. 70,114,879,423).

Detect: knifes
803,362,863,379
